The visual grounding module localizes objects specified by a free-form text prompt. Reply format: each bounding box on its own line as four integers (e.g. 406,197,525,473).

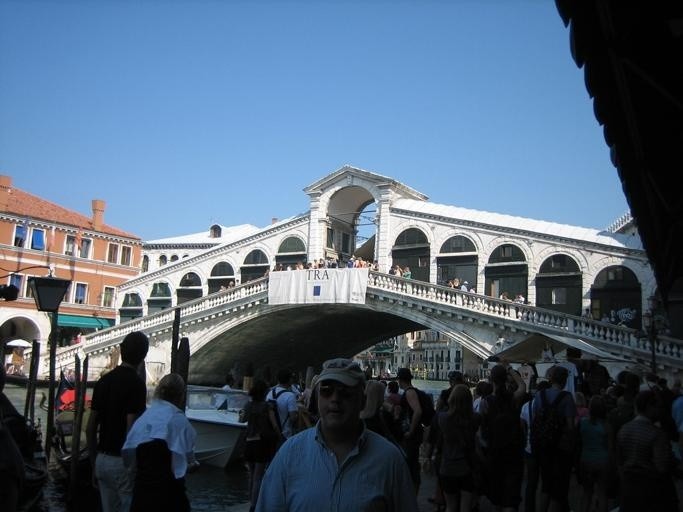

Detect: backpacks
266,387,290,443
530,388,570,458
406,387,435,426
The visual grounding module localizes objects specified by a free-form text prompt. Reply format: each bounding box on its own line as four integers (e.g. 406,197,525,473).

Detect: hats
316,359,364,386
397,368,413,378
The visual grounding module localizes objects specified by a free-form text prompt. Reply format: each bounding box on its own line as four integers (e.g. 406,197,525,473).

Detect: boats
140,380,254,473
41,362,96,415
372,371,397,382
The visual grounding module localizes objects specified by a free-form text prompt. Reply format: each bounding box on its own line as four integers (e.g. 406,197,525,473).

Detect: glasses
320,385,354,398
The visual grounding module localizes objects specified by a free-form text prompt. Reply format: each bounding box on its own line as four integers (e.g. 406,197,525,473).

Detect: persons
5,254,683,511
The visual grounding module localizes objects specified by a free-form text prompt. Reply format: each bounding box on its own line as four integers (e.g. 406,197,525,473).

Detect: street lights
26,267,70,466
646,291,662,375
